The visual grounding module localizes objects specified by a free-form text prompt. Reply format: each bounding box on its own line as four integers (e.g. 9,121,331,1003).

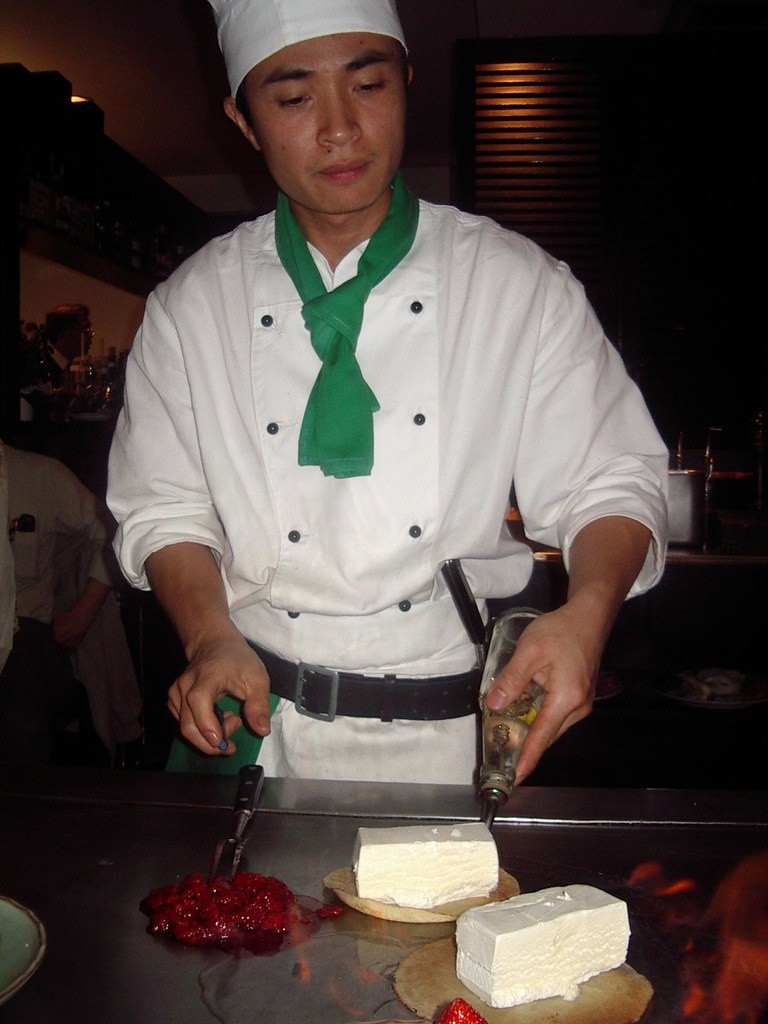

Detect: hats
207,0,408,101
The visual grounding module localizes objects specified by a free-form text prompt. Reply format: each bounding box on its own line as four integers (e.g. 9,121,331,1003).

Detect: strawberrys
436,998,488,1024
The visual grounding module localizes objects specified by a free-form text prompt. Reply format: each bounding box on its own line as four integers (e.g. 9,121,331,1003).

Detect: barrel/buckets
668,470,706,542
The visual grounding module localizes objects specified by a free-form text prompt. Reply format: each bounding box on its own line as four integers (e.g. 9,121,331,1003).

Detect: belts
245,638,484,722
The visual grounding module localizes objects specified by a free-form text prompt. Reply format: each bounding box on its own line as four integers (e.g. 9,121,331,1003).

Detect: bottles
479,606,546,831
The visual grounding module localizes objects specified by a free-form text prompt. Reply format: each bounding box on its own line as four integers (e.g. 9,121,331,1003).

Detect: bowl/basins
0,896,46,1002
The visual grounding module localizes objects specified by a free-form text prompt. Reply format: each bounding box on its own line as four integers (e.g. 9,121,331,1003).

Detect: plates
593,684,625,700
653,678,768,708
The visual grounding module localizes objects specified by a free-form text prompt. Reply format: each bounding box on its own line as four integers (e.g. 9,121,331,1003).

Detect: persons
106,0,670,784
0,304,188,790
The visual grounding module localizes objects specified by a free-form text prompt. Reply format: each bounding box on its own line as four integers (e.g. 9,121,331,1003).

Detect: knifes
209,764,264,883
